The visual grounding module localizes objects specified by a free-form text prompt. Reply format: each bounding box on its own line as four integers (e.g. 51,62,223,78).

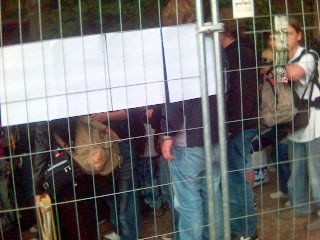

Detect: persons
129,102,171,219
262,30,295,208
90,108,144,240
261,16,320,216
208,8,266,240
0,114,125,240
158,0,225,239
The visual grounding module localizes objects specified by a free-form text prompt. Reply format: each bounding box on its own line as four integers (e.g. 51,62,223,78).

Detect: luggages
59,170,111,240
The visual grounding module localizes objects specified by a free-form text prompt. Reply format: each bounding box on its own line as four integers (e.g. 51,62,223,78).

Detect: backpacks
259,47,317,134
50,114,124,177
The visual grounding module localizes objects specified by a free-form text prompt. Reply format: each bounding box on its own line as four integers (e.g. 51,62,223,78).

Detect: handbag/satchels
42,150,78,190
250,135,270,187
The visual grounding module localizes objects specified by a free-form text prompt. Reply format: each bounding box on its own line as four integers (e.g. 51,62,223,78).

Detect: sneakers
232,231,259,240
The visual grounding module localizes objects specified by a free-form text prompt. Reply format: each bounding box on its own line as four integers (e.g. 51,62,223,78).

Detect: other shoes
280,208,303,219
285,200,291,207
305,217,320,232
140,207,164,217
270,190,288,198
